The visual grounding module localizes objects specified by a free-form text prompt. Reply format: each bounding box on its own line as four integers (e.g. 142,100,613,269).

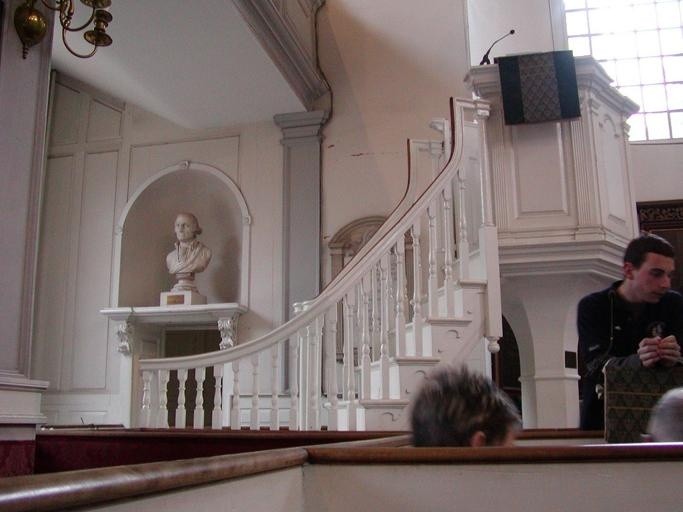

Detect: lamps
13,1,116,61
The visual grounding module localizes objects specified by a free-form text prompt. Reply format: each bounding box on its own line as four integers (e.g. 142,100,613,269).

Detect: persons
640,386,683,444
407,362,525,447
573,233,683,431
165,212,215,275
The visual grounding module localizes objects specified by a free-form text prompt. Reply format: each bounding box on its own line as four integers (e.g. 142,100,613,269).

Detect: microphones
479,30,515,65
